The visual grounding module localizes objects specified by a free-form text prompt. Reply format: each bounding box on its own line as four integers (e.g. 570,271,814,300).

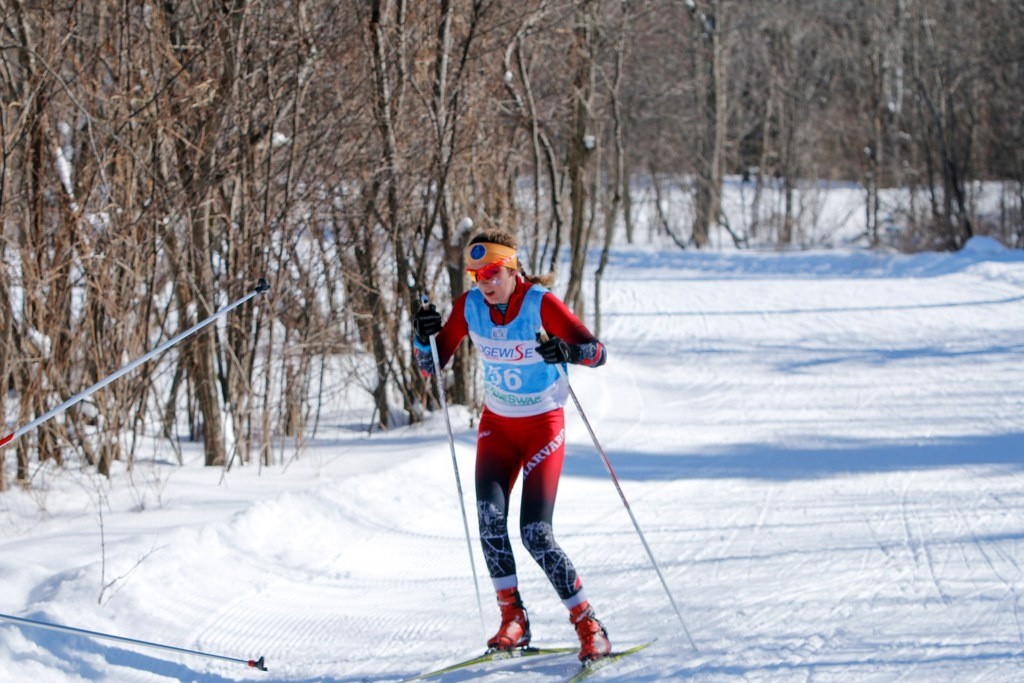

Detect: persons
413,231,612,662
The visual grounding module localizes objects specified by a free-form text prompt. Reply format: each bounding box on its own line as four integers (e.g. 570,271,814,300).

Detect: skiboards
397,634,659,680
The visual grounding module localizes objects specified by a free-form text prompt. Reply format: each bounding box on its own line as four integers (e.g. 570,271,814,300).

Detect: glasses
465,260,505,282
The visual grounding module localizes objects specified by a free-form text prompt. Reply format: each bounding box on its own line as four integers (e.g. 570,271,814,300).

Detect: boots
487,587,531,651
561,599,612,662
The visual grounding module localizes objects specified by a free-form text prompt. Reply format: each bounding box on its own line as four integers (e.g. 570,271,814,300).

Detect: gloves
535,332,581,364
412,304,442,348
